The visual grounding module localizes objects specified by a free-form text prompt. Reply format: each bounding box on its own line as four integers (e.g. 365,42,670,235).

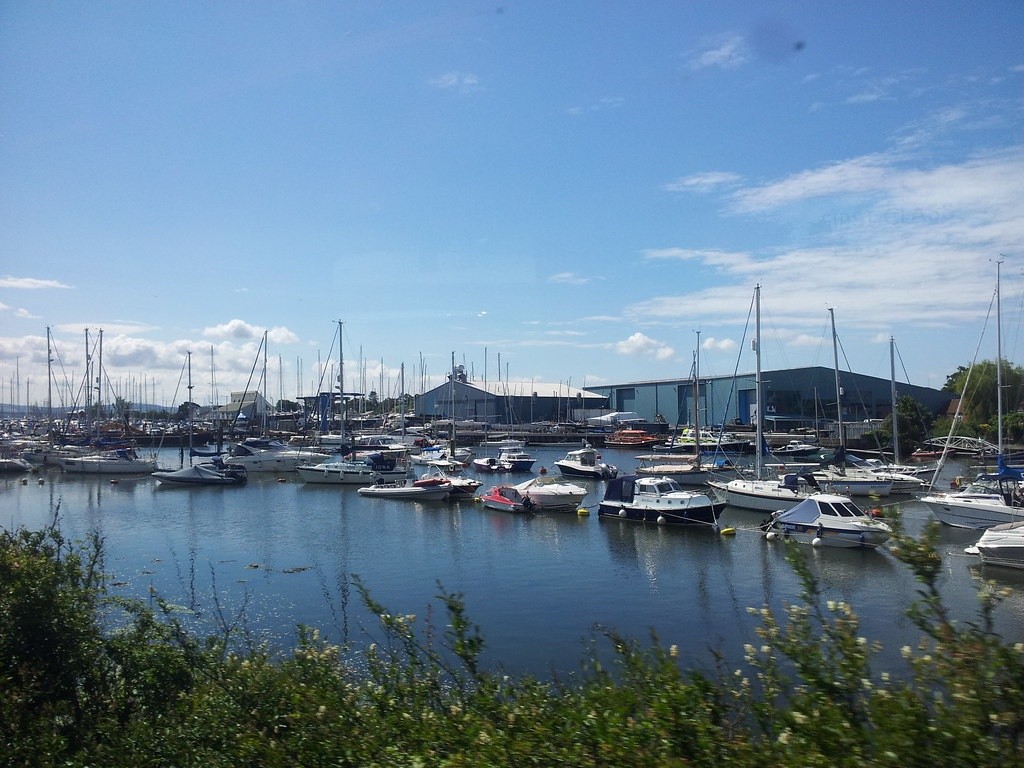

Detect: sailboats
707,282,853,512
0,317,449,486
410,391,476,467
780,307,939,499
635,329,746,484
921,261,1024,529
479,381,526,447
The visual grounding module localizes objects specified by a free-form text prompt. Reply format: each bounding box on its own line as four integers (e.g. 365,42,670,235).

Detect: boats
499,446,537,471
772,439,822,457
472,457,517,472
479,487,540,514
768,492,895,549
653,427,750,453
357,483,452,500
413,472,483,499
965,521,1024,569
511,475,588,513
603,429,661,449
597,476,726,526
555,447,619,479
